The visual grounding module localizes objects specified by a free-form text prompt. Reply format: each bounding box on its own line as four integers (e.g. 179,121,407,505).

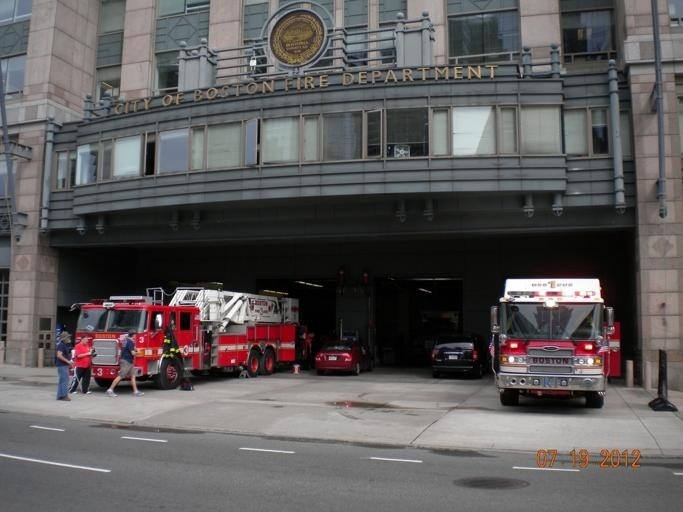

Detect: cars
313,337,373,376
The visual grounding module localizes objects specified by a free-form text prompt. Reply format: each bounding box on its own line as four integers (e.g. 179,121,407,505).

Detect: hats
129,329,138,335
59,332,72,341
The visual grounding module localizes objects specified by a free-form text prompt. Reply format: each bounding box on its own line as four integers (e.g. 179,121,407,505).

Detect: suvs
431,334,487,380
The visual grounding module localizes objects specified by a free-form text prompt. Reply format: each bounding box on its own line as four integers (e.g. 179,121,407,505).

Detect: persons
53,327,72,401
301,324,316,370
67,335,97,394
104,329,145,398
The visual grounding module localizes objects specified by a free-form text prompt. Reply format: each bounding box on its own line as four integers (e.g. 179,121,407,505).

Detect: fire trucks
412,309,460,366
68,281,299,390
488,278,615,408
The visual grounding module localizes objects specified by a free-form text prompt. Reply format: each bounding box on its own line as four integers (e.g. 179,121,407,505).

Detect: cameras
92,349,97,357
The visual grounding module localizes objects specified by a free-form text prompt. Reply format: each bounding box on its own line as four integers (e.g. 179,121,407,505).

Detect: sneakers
73,392,78,394
106,390,118,397
61,396,71,401
133,392,144,397
86,391,91,394
